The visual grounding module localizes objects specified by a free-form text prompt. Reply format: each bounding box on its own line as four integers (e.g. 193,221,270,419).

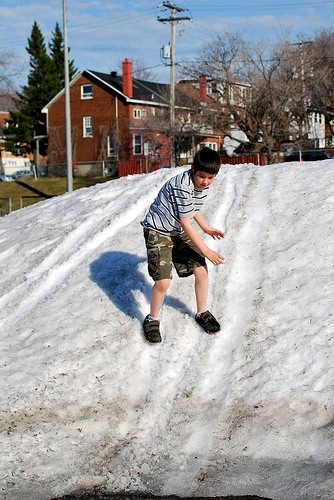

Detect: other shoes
143,314,162,344
195,310,220,334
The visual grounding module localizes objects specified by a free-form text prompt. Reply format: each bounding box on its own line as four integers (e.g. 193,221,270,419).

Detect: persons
140,147,225,343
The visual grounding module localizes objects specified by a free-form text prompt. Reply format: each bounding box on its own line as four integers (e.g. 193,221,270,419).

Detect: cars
284,150,334,162
